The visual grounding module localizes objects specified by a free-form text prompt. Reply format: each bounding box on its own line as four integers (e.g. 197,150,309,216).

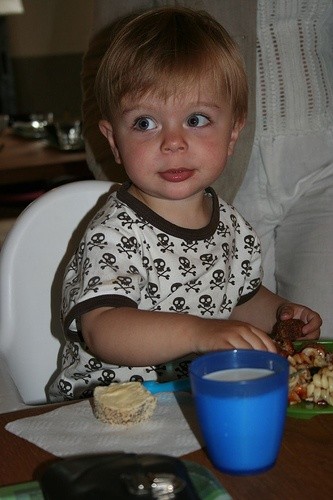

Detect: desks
0,337,333,500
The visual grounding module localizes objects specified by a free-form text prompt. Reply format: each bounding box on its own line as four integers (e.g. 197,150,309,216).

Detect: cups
188,348,289,474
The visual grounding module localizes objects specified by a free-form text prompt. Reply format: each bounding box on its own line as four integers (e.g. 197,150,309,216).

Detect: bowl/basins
43,122,85,154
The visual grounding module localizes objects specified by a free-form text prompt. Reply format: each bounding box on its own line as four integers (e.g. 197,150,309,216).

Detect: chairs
0,179,127,404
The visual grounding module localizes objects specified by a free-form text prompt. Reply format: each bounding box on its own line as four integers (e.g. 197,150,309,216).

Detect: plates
278,340,332,421
1,460,233,500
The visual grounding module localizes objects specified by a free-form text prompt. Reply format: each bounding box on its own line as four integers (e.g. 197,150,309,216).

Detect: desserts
93,381,156,422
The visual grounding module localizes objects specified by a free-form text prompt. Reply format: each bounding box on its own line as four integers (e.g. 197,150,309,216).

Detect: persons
45,3,323,404
